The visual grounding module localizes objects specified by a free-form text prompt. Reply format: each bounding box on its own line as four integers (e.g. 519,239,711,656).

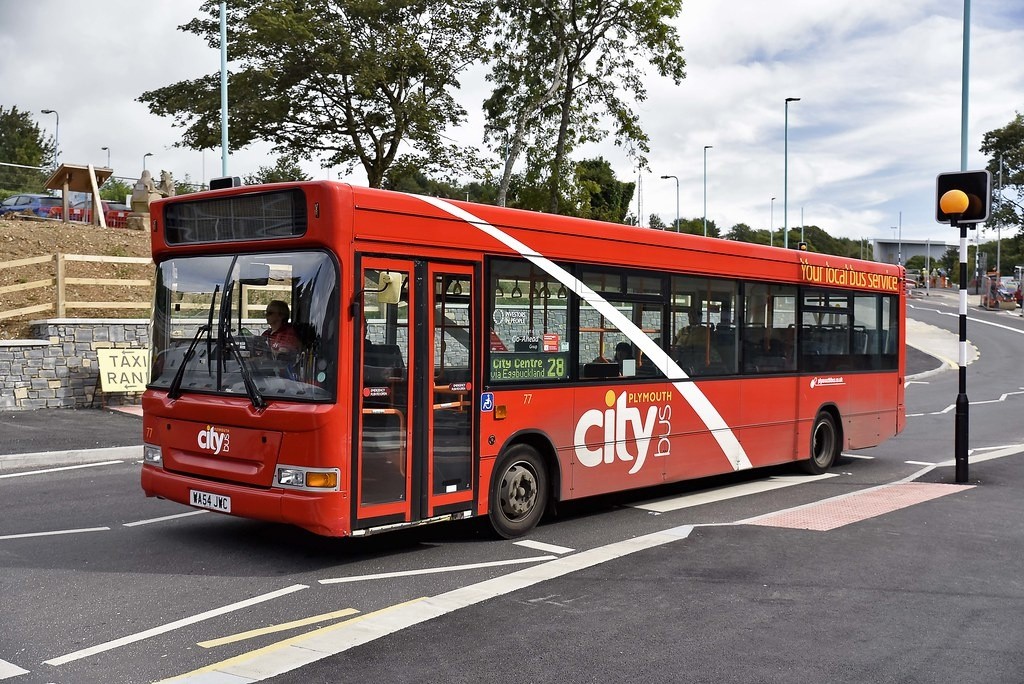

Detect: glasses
264,310,283,316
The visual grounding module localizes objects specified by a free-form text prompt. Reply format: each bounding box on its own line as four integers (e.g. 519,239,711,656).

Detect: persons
252,299,302,362
611,342,636,374
672,309,706,348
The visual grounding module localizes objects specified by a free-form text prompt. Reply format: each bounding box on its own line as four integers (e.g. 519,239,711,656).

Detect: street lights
40,109,59,170
143,152,154,171
660,175,679,233
770,197,776,247
703,146,714,238
101,146,110,168
783,96,802,249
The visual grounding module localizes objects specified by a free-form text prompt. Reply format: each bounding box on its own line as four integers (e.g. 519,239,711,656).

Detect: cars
71,199,133,213
0,194,64,218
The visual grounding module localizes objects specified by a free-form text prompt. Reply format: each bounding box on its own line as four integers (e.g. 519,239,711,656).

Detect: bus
140,175,907,538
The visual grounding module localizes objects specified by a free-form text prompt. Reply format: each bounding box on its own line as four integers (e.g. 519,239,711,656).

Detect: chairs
594,323,888,377
365,343,406,368
287,321,316,349
514,337,543,352
